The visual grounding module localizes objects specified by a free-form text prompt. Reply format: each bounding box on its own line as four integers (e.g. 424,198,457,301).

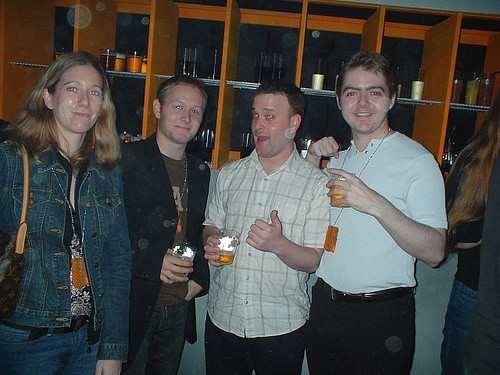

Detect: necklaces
176,150,189,234
51,167,92,288
323,126,390,253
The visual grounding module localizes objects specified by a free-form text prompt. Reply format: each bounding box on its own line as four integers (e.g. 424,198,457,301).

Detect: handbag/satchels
0,139,29,317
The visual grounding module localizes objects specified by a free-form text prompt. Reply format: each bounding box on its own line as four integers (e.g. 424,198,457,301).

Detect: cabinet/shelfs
0,0,500,182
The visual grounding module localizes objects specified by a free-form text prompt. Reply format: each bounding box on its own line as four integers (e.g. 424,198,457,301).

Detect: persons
440,96,500,375
201,79,329,375
0,51,133,375
120,75,209,375
305,50,447,375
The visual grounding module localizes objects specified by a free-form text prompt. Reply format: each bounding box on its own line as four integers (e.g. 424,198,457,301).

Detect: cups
451,70,463,103
141,56,147,74
253,52,271,84
331,173,354,208
299,138,313,159
207,49,221,81
172,240,197,277
465,71,480,105
53,46,67,61
216,229,241,265
115,53,126,72
120,133,131,144
126,51,143,73
199,128,214,163
268,54,287,85
178,46,201,79
240,133,253,158
99,48,116,71
478,73,493,106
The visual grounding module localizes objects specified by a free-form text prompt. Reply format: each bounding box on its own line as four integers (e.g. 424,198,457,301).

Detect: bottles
411,68,424,100
442,138,455,179
320,156,331,170
312,58,324,89
335,61,345,91
395,66,403,98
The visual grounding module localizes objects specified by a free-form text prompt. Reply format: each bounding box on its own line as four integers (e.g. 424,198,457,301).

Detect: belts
7,316,88,341
321,279,413,302
